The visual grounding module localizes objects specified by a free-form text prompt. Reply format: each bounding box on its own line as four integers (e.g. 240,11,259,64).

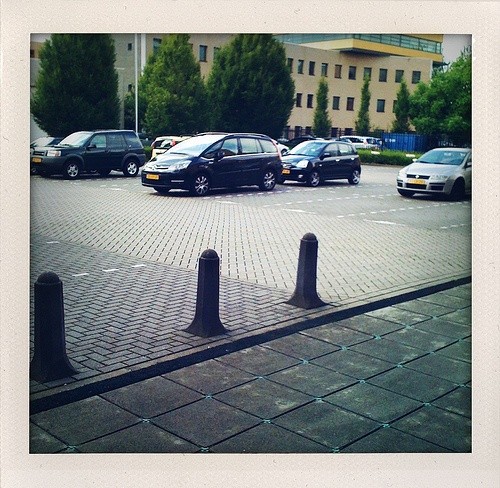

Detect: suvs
281,135,381,187
140,132,290,197
30,129,147,180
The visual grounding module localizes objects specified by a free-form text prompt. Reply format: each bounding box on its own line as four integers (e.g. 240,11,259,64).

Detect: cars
396,147,472,202
